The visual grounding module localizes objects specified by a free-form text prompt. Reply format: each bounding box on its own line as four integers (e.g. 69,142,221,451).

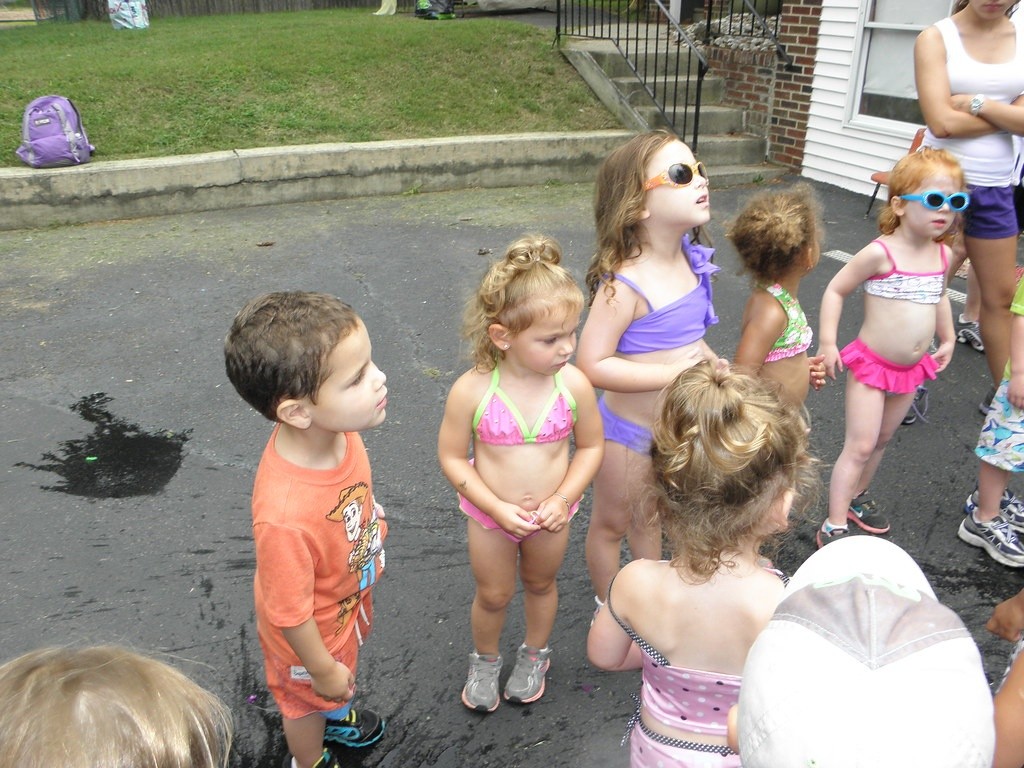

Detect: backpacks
15,95,95,168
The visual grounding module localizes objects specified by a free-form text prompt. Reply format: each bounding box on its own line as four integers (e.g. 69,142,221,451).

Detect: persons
586,360,822,768
0,646,236,768
986,588,1024,768
224,291,388,768
815,148,969,549
900,0,1024,424
727,534,996,768
957,273,1024,568
725,182,827,410
438,233,604,712
574,130,731,604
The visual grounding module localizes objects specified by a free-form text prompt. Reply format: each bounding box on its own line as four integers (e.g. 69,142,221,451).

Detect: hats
738,536,995,768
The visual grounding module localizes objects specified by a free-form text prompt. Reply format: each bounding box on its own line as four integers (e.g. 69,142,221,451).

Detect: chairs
864,127,927,218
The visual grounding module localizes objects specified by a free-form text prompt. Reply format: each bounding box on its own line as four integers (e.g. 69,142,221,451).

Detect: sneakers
323,707,385,748
847,488,891,534
816,519,850,550
927,339,937,355
963,487,1024,534
901,386,929,425
502,646,554,704
954,314,985,352
958,508,1024,567
461,653,503,713
282,746,342,768
978,388,997,415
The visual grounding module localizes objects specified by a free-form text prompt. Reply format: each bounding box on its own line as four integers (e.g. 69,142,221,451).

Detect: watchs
968,93,983,117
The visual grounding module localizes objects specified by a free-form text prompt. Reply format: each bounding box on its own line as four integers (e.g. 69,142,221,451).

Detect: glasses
898,189,970,213
642,161,708,192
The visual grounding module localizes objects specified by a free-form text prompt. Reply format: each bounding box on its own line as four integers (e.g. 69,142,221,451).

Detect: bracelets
553,492,571,514
